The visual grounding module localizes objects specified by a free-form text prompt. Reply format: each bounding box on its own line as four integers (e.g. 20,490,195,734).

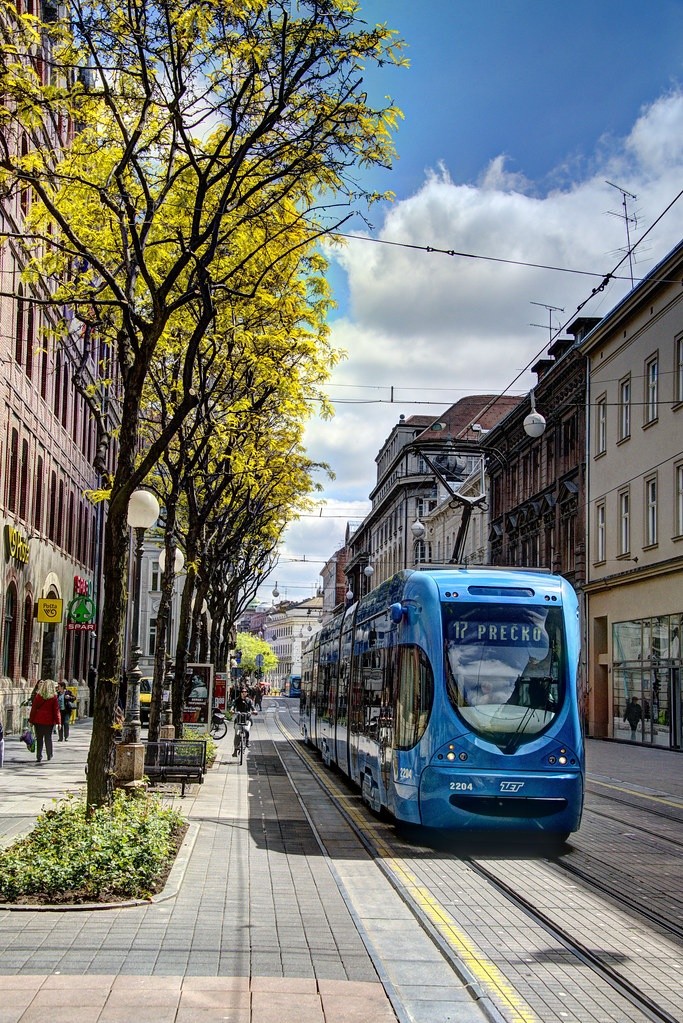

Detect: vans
138,676,154,727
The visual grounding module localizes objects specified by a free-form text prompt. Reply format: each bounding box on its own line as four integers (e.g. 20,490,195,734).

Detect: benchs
84,737,207,797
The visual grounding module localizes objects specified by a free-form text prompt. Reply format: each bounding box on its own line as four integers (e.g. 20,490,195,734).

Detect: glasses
241,691,248,693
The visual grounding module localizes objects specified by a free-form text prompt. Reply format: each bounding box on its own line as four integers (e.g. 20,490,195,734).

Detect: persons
623,696,644,739
27,678,76,763
255,687,262,711
229,688,258,757
189,672,208,697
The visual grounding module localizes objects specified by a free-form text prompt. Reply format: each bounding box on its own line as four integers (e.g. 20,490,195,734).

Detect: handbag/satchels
66,690,77,709
27,727,35,752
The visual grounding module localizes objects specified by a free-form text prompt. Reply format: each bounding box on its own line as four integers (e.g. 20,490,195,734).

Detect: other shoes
246,744,250,748
232,753,237,757
65,737,69,741
36,759,41,762
47,754,53,760
58,739,63,742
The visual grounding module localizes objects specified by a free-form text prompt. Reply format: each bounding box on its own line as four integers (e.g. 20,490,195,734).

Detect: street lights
157,545,184,739
115,492,159,794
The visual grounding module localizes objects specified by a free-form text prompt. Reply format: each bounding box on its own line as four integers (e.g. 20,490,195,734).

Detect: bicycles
210,710,228,740
229,711,257,765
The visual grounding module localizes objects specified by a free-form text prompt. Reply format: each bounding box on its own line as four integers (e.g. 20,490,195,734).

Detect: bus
280,671,301,698
297,447,586,832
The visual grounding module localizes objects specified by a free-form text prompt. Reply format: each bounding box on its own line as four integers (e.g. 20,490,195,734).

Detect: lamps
89,632,97,640
266,614,272,621
258,630,262,635
307,622,312,631
346,589,354,599
522,389,546,438
317,612,323,624
272,586,280,597
410,518,425,538
262,624,267,630
299,632,303,638
270,600,277,613
364,563,375,578
